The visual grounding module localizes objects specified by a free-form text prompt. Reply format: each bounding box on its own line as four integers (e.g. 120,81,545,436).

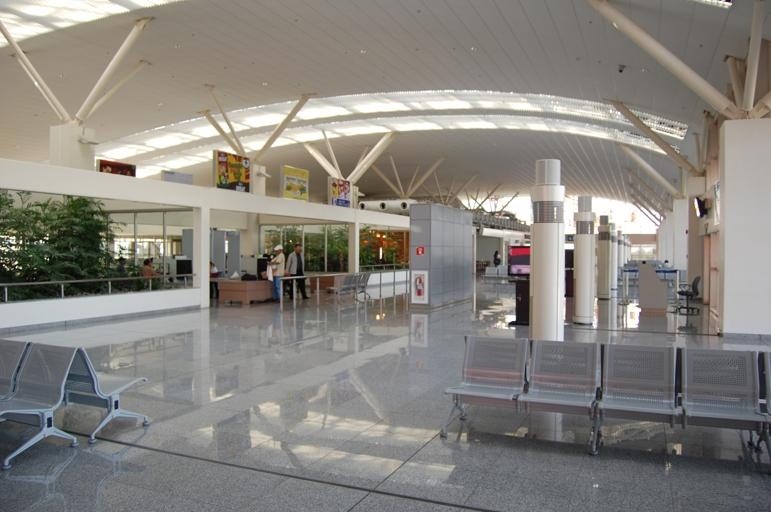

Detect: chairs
518,337,602,456
597,343,678,431
482,266,508,284
680,347,769,458
327,273,371,300
440,335,530,442
677,276,701,311
0,338,153,469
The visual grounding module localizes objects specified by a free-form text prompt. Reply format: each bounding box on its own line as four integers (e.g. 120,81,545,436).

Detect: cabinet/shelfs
508,280,529,325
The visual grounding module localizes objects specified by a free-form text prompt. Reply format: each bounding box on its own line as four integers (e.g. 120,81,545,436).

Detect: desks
310,274,345,294
622,258,679,304
217,280,273,305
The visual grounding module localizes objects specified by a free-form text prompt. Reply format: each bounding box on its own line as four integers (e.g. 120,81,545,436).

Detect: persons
267,244,286,303
210,261,220,298
494,251,502,268
144,259,160,278
286,244,310,300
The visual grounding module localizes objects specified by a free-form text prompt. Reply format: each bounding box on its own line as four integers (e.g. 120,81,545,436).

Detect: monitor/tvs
565,249,574,271
508,246,530,276
176,260,192,281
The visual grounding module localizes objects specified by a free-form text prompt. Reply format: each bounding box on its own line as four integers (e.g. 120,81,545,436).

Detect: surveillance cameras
618,65,625,72
